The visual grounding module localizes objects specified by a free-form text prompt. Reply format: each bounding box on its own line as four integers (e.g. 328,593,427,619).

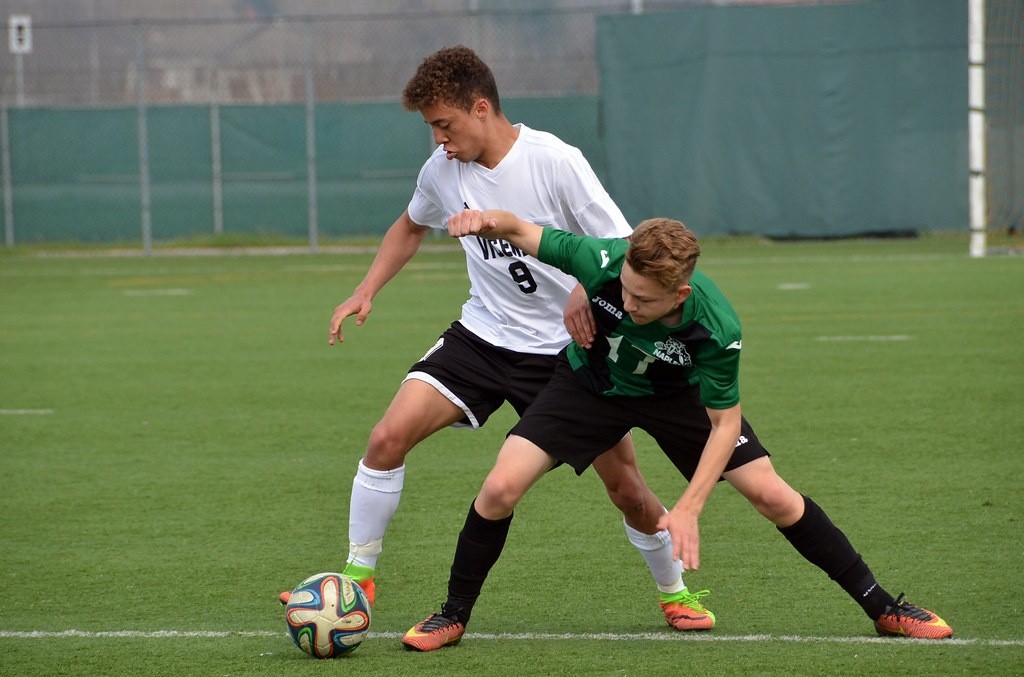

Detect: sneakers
277,563,377,612
400,612,464,653
872,591,954,641
658,586,718,632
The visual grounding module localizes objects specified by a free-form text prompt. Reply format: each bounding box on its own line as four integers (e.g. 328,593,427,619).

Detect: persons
403,207,952,643
276,43,720,633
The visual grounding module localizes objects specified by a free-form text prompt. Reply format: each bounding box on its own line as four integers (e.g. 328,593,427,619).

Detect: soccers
285,572,373,661
